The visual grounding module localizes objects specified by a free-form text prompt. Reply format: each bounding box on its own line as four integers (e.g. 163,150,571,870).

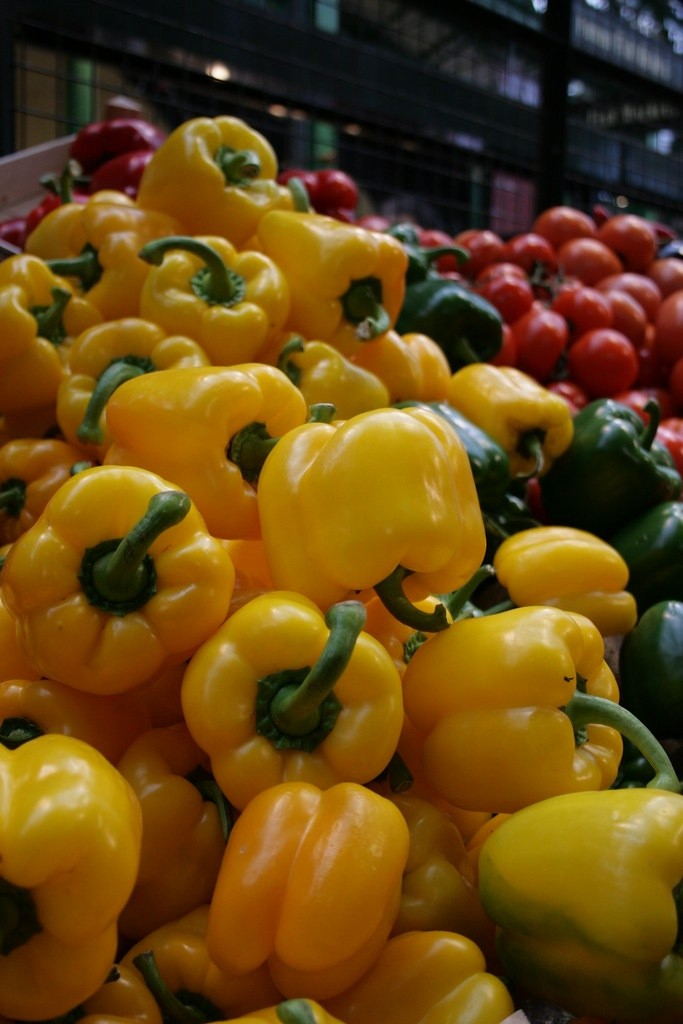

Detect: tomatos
284,171,683,472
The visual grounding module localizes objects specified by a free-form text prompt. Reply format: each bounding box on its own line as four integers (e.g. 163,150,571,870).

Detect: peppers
0,111,504,400
0,362,683,1024
